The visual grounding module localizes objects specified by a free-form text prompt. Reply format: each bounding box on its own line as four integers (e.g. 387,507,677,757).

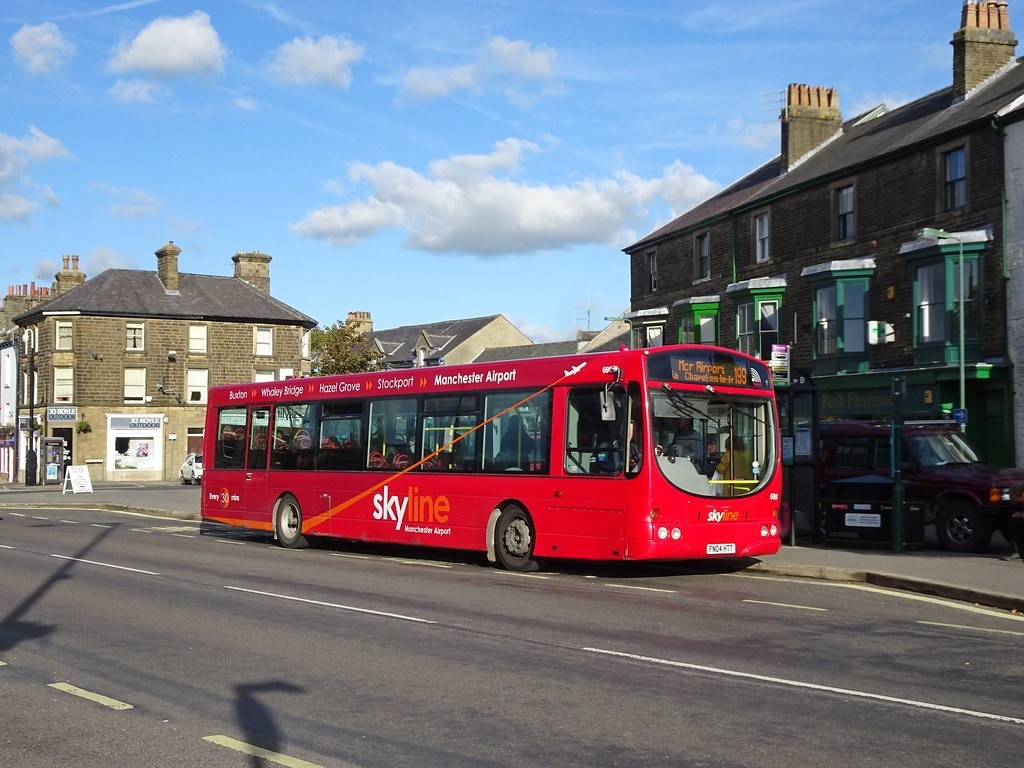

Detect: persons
500,415,534,472
716,436,756,496
276,430,284,440
613,420,641,469
672,411,704,475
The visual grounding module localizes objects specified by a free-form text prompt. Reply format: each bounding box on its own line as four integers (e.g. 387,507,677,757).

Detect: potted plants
75,422,91,433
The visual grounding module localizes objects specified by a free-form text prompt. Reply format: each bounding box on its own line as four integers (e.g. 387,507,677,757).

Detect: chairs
221,425,439,471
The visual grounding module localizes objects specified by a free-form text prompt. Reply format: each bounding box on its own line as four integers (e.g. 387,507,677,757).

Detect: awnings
219,409,311,429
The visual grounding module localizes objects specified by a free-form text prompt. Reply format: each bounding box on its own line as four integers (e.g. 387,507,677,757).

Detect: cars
179,453,203,484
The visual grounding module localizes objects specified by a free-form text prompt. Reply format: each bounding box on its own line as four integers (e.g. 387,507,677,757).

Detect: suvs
814,421,1023,553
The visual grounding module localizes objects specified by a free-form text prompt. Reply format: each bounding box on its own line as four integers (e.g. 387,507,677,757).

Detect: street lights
604,316,634,350
21,328,38,487
912,226,966,435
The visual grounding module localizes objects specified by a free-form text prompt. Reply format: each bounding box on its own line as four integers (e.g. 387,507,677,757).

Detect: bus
201,342,784,573
